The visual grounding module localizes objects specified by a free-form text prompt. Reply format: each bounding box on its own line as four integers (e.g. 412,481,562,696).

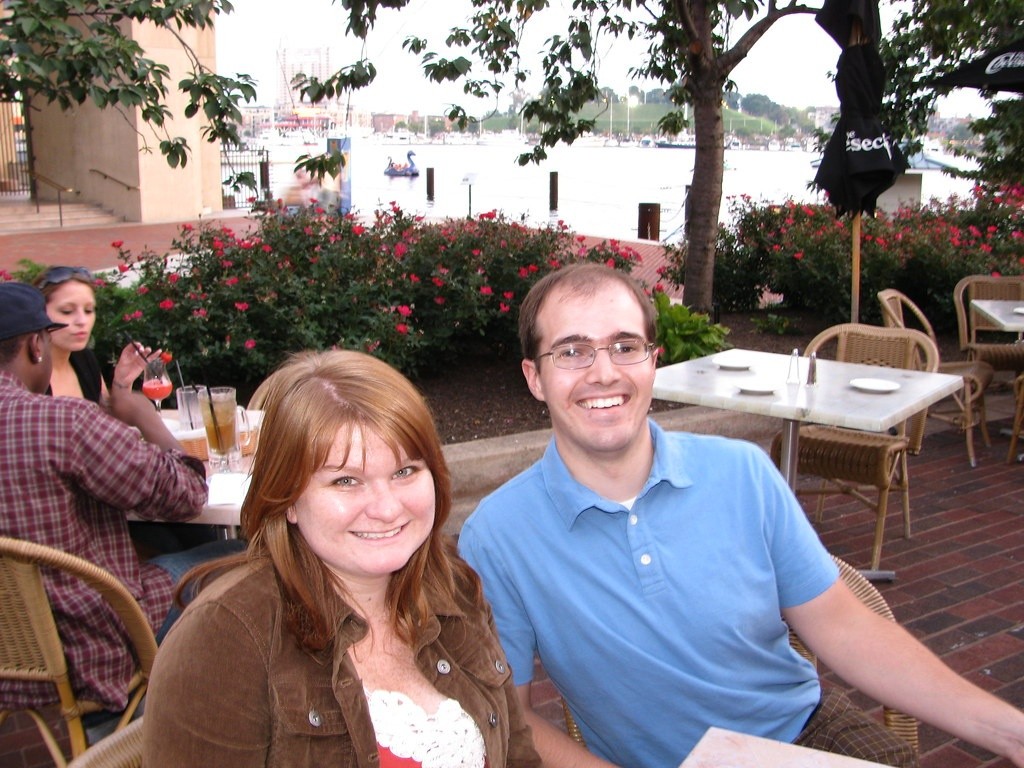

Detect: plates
1014,307,1024,313
733,382,777,394
712,357,752,369
849,378,900,393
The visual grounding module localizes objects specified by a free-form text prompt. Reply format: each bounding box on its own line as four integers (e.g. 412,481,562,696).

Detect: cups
177,385,206,434
198,387,251,472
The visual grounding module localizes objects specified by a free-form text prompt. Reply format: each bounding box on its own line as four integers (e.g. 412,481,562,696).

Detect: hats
0,282,70,341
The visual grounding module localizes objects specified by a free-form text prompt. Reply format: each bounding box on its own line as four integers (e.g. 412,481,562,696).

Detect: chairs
878,274,1024,467
0,537,157,768
770,323,940,572
564,555,918,759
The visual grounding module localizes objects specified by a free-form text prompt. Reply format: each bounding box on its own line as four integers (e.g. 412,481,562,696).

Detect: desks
651,349,964,583
968,298,1024,462
125,410,269,541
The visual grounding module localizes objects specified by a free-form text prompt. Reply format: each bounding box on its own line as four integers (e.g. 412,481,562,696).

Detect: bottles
805,352,817,386
787,348,801,385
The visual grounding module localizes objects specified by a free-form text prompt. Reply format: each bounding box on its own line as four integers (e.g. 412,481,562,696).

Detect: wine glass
142,358,172,416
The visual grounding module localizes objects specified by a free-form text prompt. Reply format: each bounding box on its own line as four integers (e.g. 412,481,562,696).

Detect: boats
384,150,419,177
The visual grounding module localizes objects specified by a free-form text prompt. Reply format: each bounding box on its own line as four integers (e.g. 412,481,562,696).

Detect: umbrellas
927,37,1024,92
817,0,911,323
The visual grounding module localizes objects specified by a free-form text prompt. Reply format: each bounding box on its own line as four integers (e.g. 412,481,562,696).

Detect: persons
143,352,543,767
394,162,408,172
0,282,252,717
30,264,162,408
457,264,1024,766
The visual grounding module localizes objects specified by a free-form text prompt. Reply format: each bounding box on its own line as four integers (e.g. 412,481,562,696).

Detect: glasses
533,340,655,370
39,265,94,290
